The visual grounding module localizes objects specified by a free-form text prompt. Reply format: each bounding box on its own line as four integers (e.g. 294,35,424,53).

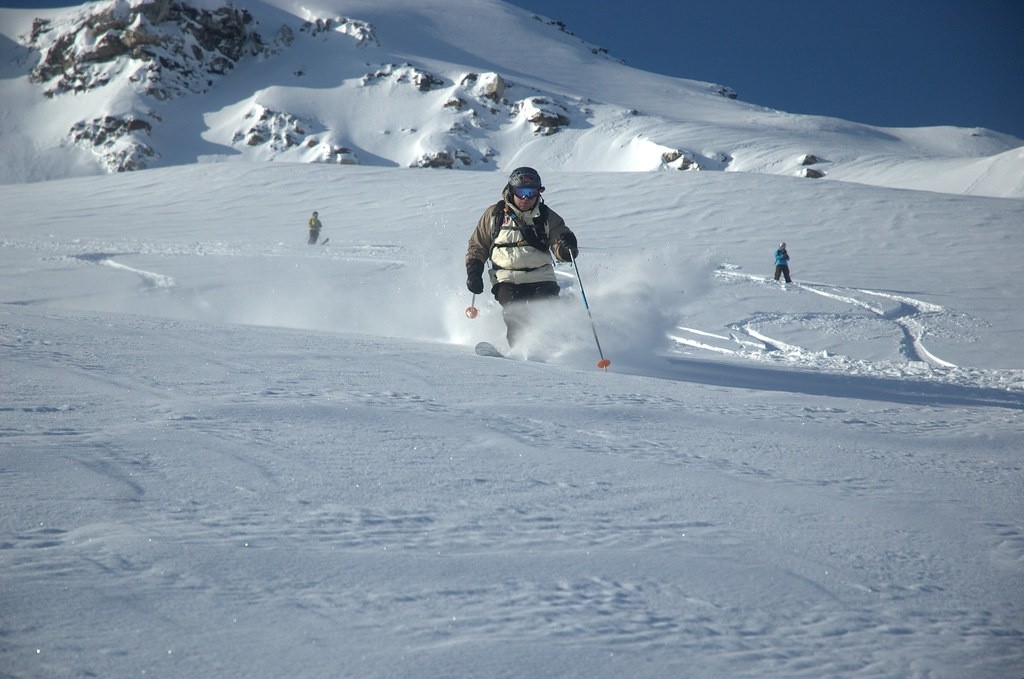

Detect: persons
308,212,322,246
773,241,792,283
464,167,578,362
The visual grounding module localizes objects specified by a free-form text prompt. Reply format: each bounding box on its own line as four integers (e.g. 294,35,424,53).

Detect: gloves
559,232,579,263
465,259,484,294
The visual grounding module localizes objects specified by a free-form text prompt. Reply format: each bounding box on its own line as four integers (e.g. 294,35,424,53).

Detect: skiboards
305,236,330,245
473,341,553,364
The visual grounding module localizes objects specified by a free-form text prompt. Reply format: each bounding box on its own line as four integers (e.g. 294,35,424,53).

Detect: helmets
508,167,542,187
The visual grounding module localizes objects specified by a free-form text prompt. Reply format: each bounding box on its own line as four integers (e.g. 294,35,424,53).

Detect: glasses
510,187,539,199
